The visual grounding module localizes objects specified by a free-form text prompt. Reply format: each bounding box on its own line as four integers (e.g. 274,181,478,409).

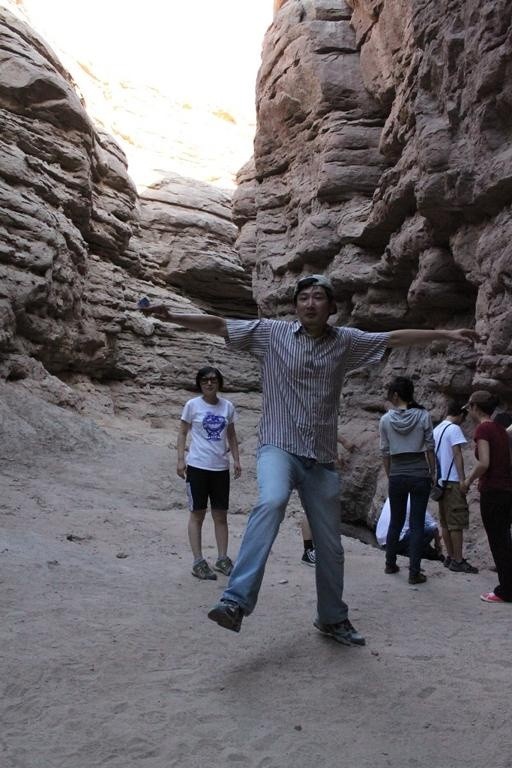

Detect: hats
461,390,492,409
292,274,335,307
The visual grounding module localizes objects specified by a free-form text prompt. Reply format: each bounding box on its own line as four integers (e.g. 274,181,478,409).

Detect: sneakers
384,564,400,574
214,556,234,576
191,560,217,581
207,598,245,633
300,547,316,566
480,591,505,603
408,572,427,585
312,617,366,646
448,558,479,574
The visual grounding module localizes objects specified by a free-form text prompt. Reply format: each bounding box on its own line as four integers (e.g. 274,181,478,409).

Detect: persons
176,367,242,581
300,431,358,567
376,488,445,562
432,402,479,574
494,412,512,436
136,275,481,648
379,377,436,584
462,391,512,603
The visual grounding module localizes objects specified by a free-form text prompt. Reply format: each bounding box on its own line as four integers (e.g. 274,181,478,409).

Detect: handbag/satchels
429,423,455,501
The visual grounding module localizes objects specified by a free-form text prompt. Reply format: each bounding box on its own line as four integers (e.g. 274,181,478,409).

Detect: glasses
200,377,219,384
